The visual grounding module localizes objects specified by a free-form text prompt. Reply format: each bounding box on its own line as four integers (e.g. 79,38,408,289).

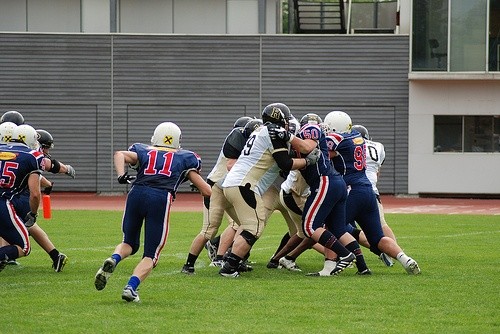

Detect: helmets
0,110,55,159
151,121,182,149
234,103,369,141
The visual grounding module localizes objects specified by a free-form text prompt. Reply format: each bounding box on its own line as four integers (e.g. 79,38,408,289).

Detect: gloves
118,172,135,184
305,147,321,166
42,181,54,194
190,182,200,192
24,211,35,226
66,164,77,181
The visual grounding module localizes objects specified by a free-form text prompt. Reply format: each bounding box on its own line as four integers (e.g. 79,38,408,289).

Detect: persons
94,122,212,304
0,110,75,272
181,102,421,279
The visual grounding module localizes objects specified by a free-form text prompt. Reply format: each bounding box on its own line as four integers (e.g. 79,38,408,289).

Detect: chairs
429,39,448,69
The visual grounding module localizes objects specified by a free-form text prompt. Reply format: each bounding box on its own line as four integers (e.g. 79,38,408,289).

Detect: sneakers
181,234,256,278
354,268,371,275
320,257,339,276
380,253,394,266
330,252,356,275
122,286,139,303
268,257,286,269
94,258,116,291
54,253,68,273
404,257,420,275
279,256,302,270
